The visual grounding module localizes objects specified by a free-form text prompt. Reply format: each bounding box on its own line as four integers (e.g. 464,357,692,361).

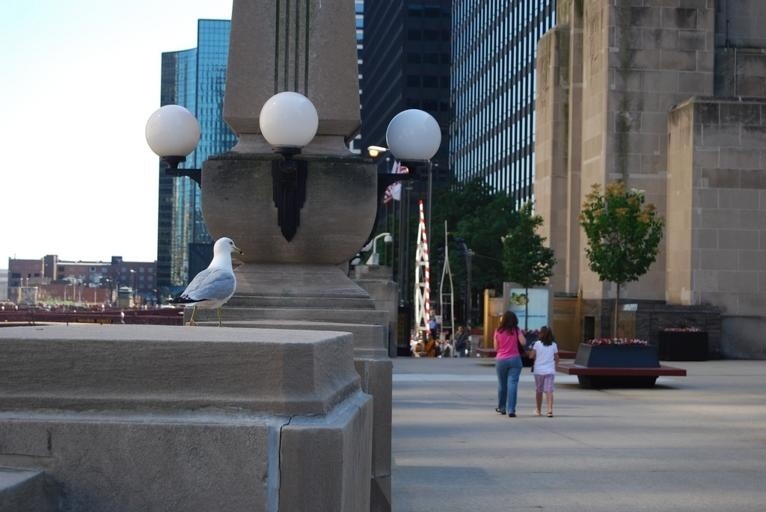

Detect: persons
493,310,528,417
525,324,560,418
408,307,471,358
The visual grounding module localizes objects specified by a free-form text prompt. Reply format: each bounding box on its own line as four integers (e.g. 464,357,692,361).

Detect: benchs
476,348,574,358
553,363,687,377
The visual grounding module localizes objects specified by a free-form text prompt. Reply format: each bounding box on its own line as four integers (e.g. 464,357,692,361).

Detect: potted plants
499,199,558,367
574,180,660,390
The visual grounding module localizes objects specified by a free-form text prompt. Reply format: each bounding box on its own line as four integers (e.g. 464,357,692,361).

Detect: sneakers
533,409,553,417
495,408,516,418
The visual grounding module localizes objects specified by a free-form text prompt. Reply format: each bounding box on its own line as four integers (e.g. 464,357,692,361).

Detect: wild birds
165,237,245,327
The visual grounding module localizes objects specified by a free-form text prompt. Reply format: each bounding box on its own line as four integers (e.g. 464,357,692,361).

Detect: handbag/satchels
518,342,534,368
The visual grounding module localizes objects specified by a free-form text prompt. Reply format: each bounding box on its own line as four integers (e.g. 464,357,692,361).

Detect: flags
382,159,409,203
